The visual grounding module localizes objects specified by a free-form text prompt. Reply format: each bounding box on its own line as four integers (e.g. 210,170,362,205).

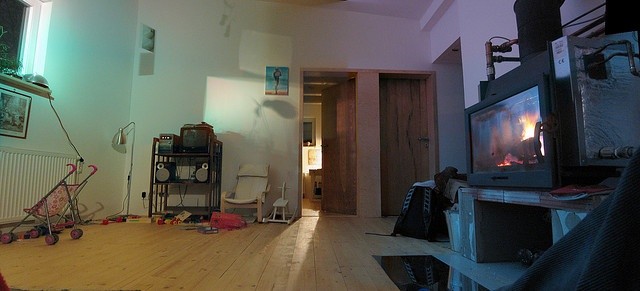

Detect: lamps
106,122,136,221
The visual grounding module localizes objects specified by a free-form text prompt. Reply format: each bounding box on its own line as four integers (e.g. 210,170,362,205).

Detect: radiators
1,146,82,226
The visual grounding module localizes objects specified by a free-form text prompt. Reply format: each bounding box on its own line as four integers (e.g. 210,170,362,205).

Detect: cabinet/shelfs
148,138,223,223
456,188,609,263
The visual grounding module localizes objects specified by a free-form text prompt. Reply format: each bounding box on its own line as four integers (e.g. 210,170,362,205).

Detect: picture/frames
0,88,32,139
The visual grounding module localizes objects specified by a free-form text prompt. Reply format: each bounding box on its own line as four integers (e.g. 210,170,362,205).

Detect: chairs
221,164,271,222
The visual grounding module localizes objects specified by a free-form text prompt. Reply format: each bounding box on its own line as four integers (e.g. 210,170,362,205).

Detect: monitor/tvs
180,126,213,154
463,72,579,190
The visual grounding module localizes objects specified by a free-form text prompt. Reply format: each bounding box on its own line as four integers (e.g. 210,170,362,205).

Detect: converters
142,192,146,198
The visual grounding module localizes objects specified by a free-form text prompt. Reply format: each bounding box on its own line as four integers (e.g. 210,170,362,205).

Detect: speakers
196,162,210,183
156,161,176,183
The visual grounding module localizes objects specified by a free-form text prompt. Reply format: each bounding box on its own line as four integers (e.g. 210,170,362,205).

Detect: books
548,184,615,201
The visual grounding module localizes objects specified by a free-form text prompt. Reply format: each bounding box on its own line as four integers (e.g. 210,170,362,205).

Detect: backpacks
391,185,453,243
